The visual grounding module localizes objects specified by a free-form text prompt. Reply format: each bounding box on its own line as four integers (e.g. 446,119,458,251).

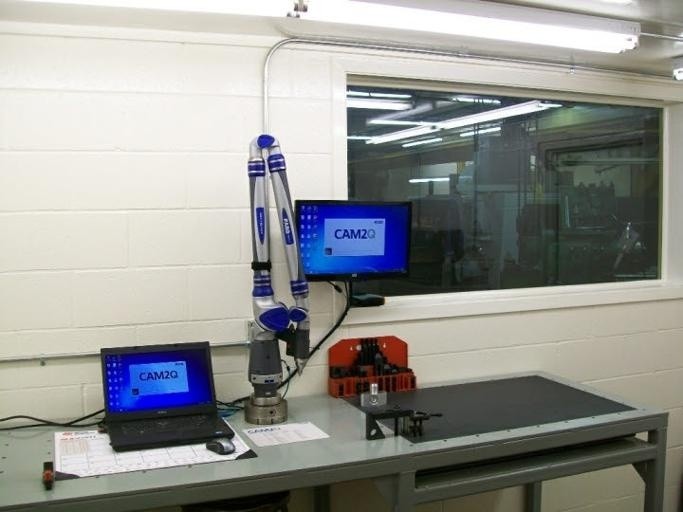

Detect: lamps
293,0,643,57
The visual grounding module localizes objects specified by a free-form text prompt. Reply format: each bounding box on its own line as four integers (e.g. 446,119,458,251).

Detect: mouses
206,436,235,455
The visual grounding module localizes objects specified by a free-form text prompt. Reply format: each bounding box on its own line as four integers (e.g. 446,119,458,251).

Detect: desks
1,372,668,511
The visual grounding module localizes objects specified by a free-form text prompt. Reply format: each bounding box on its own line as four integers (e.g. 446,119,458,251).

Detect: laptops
100,341,235,452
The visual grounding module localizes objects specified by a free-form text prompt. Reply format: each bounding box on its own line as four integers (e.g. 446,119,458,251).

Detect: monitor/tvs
294,199,413,283
